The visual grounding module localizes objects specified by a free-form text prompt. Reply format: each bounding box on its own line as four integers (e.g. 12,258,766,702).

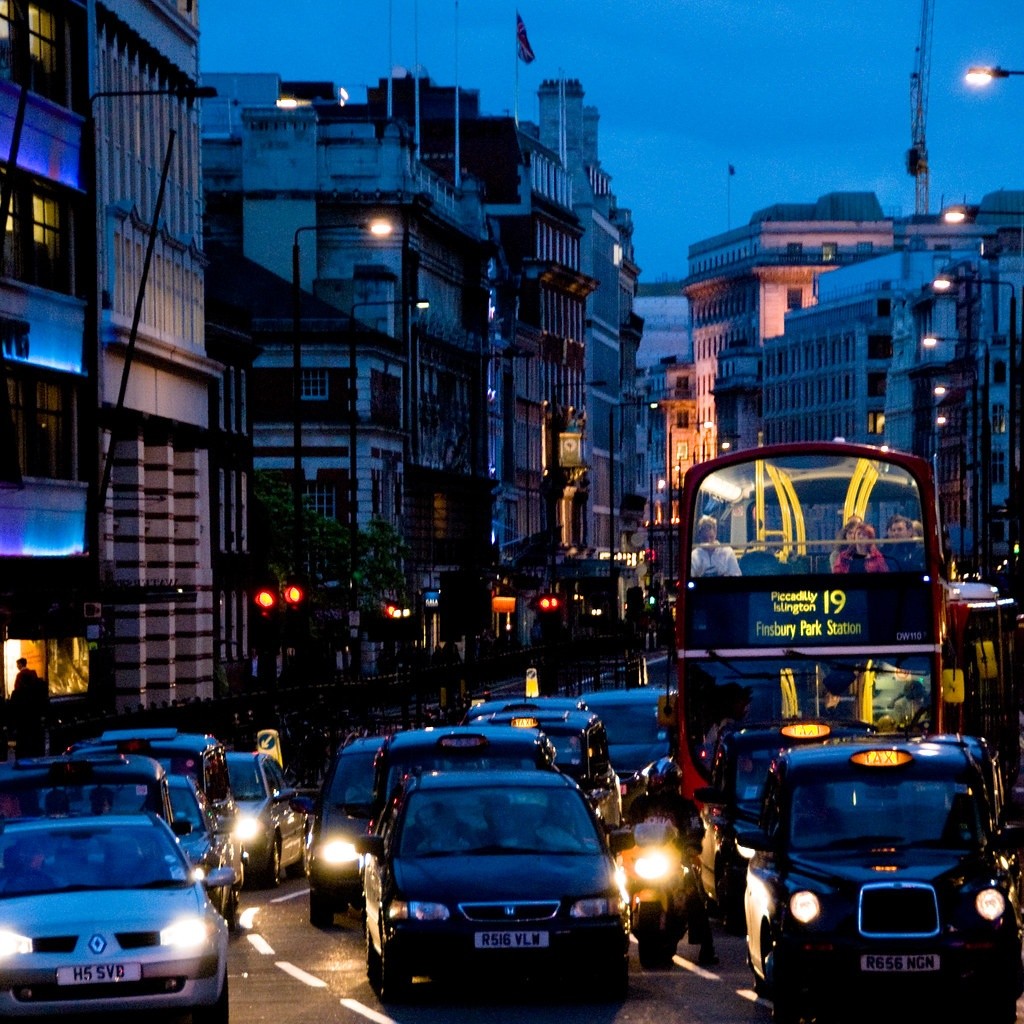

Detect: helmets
648,761,682,798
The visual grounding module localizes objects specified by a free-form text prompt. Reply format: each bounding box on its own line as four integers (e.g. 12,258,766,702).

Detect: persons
111,829,171,887
820,690,850,719
691,515,742,577
879,515,926,573
479,791,542,854
829,520,888,575
8,659,46,758
894,682,926,731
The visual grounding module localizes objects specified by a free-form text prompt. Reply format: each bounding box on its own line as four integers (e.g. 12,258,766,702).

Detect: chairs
737,551,831,574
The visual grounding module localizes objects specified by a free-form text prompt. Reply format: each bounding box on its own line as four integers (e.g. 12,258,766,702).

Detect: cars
0,435,1024,1024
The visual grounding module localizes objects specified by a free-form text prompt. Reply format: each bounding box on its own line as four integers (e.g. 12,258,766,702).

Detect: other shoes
692,851,719,966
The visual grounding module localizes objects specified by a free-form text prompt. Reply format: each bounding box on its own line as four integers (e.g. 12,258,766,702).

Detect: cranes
904,0,936,215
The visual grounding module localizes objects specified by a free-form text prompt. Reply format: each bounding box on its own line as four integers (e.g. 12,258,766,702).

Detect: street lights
608,401,661,571
934,415,964,553
932,385,979,549
343,296,433,529
479,350,535,479
552,380,605,470
930,275,1017,546
287,218,396,589
75,84,219,579
919,335,996,553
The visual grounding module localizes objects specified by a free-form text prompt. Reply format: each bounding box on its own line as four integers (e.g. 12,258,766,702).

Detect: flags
515,10,535,63
